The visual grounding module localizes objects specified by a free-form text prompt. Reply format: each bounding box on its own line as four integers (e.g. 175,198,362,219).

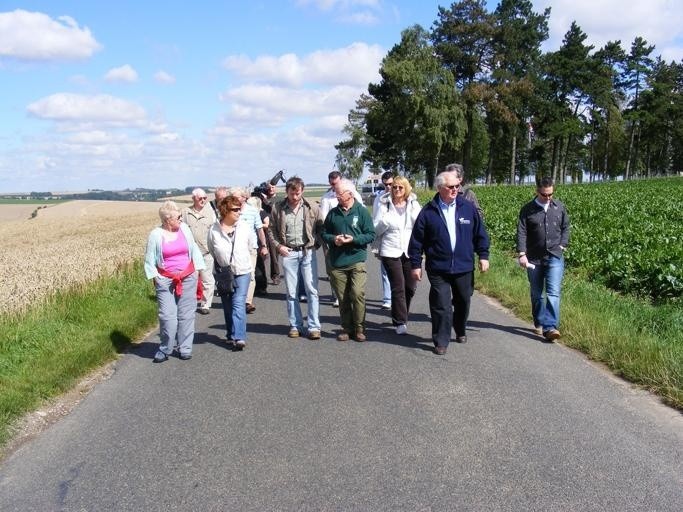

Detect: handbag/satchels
215,266,236,298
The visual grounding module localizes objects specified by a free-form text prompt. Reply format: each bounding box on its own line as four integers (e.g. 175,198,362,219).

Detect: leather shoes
434,345,447,355
457,334,467,344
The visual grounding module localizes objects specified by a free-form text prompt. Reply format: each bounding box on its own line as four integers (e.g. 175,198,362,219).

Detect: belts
284,244,315,251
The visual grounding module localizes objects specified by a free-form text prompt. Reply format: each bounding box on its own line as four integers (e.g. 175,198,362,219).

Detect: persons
517,178,570,341
144,163,490,360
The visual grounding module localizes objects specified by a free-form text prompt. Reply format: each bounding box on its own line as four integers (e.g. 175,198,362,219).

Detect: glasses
540,192,554,198
392,186,403,189
177,214,183,220
229,208,241,213
449,185,460,189
384,183,391,186
197,197,207,200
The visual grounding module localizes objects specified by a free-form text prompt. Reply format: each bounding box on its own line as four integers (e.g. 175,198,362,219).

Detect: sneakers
355,332,366,343
338,329,351,341
246,304,256,313
396,324,407,335
233,339,246,350
154,350,169,362
382,302,391,309
178,353,192,360
308,330,320,340
534,326,542,333
544,329,561,341
198,309,209,314
287,329,300,338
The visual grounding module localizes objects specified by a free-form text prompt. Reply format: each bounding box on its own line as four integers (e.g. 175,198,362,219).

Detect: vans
361,175,381,200
